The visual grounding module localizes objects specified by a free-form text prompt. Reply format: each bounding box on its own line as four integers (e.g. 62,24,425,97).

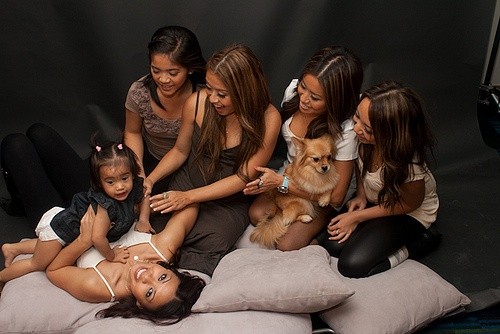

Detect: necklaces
372,166,380,173
225,127,229,134
125,255,153,294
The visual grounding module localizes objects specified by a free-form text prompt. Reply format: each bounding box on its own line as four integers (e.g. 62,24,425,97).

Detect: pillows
0,225,471,334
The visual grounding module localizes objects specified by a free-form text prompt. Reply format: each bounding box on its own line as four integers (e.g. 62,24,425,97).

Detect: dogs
247,130,340,250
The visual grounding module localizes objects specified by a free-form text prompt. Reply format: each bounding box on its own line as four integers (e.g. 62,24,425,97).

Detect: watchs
277,176,290,194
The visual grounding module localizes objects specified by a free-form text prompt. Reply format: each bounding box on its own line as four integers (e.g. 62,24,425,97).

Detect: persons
0,25,439,327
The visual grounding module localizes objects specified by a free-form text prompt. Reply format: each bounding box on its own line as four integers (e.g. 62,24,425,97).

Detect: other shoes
405,228,437,261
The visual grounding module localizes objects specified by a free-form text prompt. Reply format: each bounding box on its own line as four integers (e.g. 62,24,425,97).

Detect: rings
258,177,264,187
163,192,167,199
335,231,339,234
